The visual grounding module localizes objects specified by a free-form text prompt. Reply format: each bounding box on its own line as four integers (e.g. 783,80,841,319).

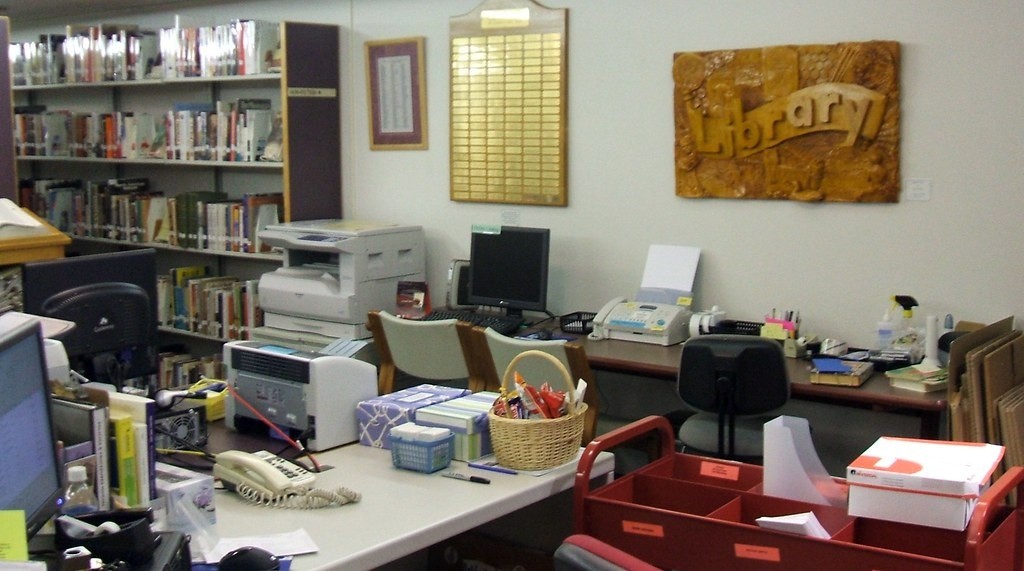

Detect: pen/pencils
468,463,518,475
442,474,491,485
773,308,776,319
784,310,801,324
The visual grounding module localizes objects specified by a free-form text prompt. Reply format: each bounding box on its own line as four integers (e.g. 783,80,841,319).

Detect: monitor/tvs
0,311,66,571
468,223,551,317
21,248,162,392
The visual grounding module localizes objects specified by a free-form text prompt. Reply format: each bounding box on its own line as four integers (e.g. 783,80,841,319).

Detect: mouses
218,546,281,571
539,328,553,340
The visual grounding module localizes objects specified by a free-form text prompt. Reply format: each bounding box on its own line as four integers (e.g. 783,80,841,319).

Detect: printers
257,220,429,340
593,297,694,346
223,340,380,453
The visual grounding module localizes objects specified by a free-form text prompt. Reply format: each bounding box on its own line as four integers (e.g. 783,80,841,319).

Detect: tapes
820,338,848,358
94,521,120,536
798,337,807,347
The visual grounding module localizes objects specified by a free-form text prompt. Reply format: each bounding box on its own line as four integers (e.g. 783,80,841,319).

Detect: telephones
213,449,315,502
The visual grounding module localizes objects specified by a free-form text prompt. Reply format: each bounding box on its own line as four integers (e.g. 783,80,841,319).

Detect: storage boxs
845,435,1006,532
414,390,502,463
356,383,472,451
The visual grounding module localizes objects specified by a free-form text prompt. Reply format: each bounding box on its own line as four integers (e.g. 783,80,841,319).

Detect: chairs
41,283,152,385
366,310,631,448
677,334,813,465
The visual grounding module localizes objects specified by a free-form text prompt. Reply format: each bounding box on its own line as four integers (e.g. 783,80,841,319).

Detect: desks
155,417,614,571
361,302,950,440
0,206,72,265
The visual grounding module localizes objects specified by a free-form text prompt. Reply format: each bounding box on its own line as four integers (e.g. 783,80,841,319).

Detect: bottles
58,464,100,518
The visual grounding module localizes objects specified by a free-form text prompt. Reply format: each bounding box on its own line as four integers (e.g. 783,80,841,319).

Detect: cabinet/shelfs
14,21,344,343
570,415,1024,570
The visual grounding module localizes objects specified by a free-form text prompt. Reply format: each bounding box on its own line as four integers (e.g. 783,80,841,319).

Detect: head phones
151,388,207,411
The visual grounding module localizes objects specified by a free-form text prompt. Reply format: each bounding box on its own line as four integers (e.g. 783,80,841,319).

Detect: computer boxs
144,395,208,452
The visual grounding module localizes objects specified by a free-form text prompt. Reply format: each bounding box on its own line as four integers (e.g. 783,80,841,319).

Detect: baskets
388,431,456,473
487,350,588,469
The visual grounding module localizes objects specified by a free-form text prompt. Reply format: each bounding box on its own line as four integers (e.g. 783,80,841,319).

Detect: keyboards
423,309,526,337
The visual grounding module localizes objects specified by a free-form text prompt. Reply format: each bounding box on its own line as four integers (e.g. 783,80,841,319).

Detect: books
12,14,283,85
13,98,284,163
159,351,223,389
19,177,285,253
809,362,875,387
156,266,264,341
885,364,950,393
49,389,156,510
